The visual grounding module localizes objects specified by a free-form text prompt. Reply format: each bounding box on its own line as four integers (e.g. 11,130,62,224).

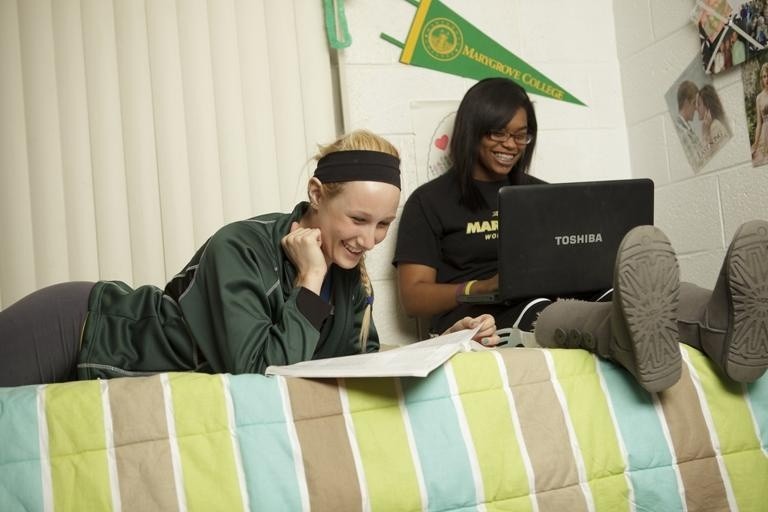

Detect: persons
391,78,768,394
710,0,768,74
750,61,768,164
696,83,731,158
0,128,500,387
675,81,714,169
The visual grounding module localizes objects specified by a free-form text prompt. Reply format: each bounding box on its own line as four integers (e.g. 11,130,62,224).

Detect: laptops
456,179,655,305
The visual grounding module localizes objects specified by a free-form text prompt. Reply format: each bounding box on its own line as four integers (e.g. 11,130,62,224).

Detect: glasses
482,131,534,145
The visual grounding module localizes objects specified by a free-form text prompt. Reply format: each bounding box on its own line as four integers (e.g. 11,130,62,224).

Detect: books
265,323,499,381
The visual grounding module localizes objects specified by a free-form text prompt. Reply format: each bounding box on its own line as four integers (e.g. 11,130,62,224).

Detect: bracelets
455,281,464,304
465,279,477,295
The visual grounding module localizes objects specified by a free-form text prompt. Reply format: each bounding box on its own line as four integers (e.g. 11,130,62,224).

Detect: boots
534,220,685,394
675,217,768,385
